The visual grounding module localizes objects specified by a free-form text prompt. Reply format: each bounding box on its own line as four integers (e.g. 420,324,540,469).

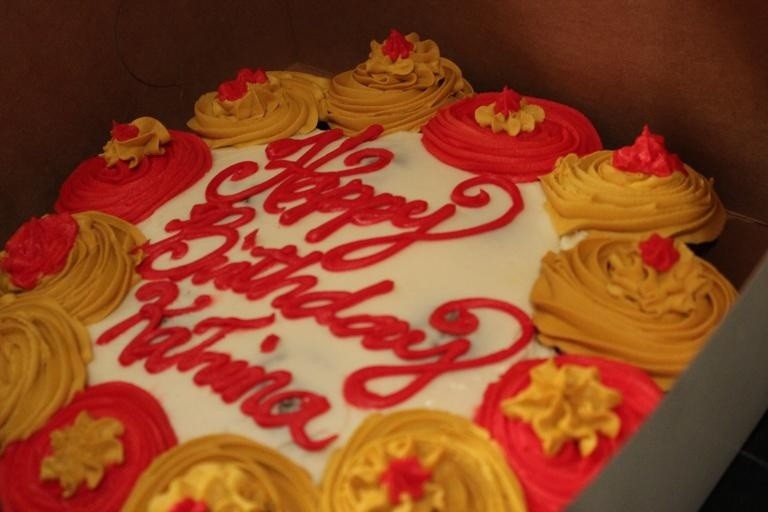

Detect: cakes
0,28,741,512
